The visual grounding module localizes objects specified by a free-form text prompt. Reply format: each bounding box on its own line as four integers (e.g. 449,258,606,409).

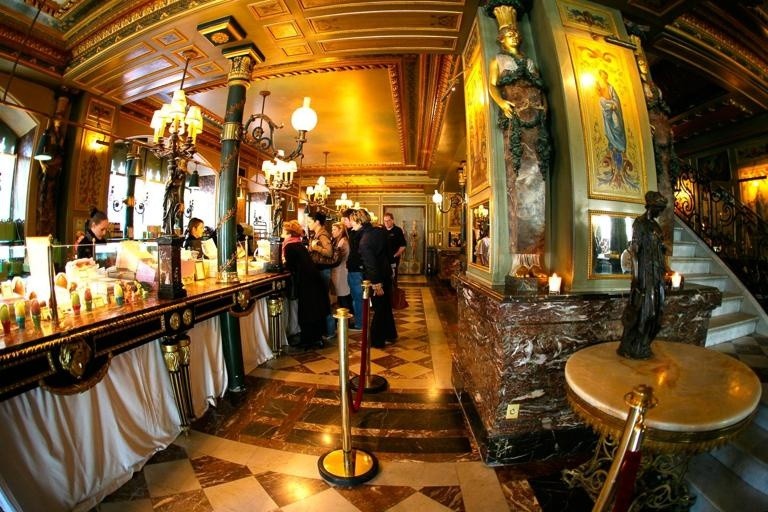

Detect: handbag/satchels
307,232,343,269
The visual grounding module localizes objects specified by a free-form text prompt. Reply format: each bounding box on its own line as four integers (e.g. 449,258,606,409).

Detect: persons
592,69,640,193
487,5,552,277
449,220,490,269
72,206,113,270
236,224,254,257
279,207,409,350
617,191,667,361
399,219,421,261
183,217,217,260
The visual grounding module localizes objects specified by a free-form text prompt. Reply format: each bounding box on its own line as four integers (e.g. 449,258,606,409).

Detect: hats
283,219,303,236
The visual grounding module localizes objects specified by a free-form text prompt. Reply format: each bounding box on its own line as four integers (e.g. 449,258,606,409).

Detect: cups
548,276,562,295
671,275,682,288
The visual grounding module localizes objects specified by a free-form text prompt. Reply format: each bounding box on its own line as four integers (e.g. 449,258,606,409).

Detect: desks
562,337,764,511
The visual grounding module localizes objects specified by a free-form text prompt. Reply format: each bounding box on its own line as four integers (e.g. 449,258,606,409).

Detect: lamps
432,189,464,214
34,113,56,164
260,148,298,194
149,49,206,158
304,151,331,208
189,163,201,190
240,95,319,165
